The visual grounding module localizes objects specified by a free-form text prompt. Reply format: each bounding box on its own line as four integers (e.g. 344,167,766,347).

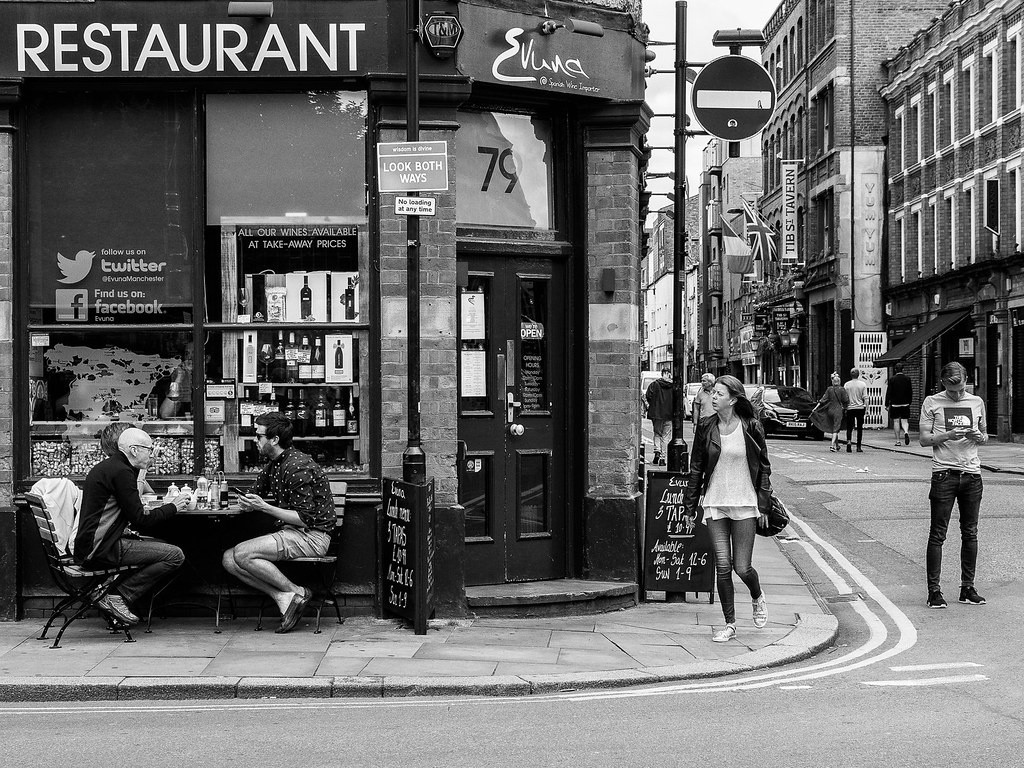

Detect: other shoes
653,452,660,464
304,588,312,604
905,433,909,445
275,593,308,633
836,446,840,450
659,459,666,466
894,442,901,446
830,447,836,452
712,623,736,642
752,589,768,629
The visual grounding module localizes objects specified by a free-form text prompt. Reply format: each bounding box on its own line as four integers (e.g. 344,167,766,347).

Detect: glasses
129,445,154,455
256,433,266,440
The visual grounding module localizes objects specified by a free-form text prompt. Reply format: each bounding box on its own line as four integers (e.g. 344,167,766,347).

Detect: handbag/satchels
756,496,790,537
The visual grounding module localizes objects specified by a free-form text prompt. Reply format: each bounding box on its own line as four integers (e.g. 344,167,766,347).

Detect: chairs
25,477,349,648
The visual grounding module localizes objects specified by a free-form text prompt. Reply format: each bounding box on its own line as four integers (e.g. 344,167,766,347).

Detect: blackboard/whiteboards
640,469,716,605
381,476,437,635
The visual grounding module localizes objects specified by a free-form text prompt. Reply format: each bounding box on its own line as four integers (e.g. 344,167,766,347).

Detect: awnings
873,309,972,368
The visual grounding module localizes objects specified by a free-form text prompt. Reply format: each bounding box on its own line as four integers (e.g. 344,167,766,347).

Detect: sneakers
94,590,139,625
926,591,948,608
958,586,986,604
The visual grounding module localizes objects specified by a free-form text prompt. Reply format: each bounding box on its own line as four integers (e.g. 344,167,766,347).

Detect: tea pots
166,482,199,510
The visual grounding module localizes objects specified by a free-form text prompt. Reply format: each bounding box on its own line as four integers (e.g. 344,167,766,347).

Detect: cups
162,496,176,505
141,493,158,504
145,397,157,419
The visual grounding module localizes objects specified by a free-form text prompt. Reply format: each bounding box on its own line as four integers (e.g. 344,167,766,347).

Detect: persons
51,370,83,421
919,363,989,609
68,423,191,624
693,373,717,434
844,368,869,452
159,341,216,420
685,376,772,643
808,373,850,453
646,367,674,466
222,412,337,633
885,363,912,446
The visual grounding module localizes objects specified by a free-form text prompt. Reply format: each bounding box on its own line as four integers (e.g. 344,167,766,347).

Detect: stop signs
690,54,778,142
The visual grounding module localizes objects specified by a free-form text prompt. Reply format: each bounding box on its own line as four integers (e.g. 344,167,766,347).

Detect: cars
683,382,702,420
744,384,825,442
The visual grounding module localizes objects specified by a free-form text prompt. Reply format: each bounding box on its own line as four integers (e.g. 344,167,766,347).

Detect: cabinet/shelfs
220,216,370,472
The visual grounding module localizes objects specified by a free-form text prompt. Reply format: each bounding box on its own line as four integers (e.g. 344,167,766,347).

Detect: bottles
333,339,344,375
345,277,354,319
300,276,311,319
245,335,255,377
274,330,326,383
197,473,229,511
238,387,359,437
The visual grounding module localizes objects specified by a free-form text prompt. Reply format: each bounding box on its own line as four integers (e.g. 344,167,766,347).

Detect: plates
164,415,193,420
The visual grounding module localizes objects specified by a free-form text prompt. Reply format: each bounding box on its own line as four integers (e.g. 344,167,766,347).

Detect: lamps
645,172,675,180
651,193,675,201
653,113,690,125
645,66,697,83
541,17,604,37
603,268,615,294
647,210,674,219
457,262,468,287
748,322,802,350
227,2,274,21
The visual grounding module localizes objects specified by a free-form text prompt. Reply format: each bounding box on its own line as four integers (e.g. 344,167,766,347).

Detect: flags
722,219,754,274
743,200,779,262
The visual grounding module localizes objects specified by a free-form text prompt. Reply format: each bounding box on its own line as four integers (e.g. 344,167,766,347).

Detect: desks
144,500,245,634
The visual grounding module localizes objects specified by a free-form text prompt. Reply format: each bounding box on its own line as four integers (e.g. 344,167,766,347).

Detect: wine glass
167,381,181,421
237,288,252,315
258,344,277,384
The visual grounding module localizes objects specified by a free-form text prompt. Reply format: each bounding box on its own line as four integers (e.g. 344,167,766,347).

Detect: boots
857,445,863,452
847,440,852,452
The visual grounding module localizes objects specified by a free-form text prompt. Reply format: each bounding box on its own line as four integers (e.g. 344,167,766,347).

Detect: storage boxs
326,335,353,383
264,271,359,323
243,331,257,383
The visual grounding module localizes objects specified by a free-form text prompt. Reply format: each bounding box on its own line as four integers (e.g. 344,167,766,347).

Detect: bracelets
137,480,145,484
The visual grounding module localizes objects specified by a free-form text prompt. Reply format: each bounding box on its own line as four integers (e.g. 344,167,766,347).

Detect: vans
641,372,662,394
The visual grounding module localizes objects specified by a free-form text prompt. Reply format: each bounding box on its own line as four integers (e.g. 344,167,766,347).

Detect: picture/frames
996,364,1002,386
974,366,979,387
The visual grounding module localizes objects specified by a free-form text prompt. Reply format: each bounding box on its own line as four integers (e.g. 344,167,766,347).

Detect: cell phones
229,486,246,497
